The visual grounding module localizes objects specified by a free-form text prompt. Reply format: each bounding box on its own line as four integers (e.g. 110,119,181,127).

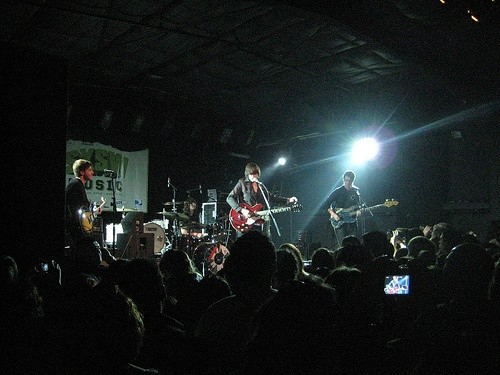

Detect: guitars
229,202,302,233
328,199,399,227
78,197,106,235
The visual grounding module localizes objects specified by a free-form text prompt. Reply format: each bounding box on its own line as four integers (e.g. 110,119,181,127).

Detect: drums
193,240,232,273
144,222,165,252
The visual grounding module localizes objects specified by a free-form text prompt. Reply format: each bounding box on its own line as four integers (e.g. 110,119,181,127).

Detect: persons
0,223,499,375
322,170,364,248
64,158,102,247
226,162,299,246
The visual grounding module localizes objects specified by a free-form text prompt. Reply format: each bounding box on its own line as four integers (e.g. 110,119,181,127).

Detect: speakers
116,233,154,260
121,211,145,233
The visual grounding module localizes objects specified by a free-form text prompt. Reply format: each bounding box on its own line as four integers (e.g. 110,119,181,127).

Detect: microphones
252,177,262,185
199,182,202,194
104,169,114,173
168,178,171,187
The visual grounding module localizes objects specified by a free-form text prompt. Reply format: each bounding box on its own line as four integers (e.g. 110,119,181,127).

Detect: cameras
384,274,411,296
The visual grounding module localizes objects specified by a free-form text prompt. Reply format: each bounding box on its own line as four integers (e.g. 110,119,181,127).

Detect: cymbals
161,198,189,224
